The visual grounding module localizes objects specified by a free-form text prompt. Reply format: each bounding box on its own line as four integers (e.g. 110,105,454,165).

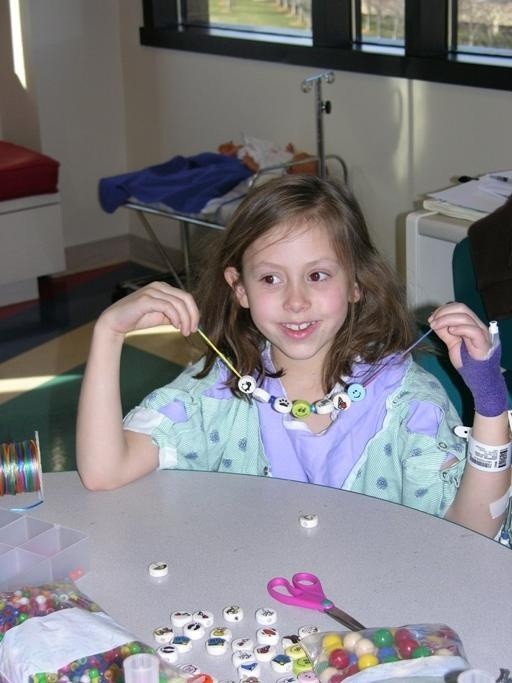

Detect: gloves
451,339,509,416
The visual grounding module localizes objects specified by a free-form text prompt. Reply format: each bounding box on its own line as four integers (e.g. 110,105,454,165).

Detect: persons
75,171,511,539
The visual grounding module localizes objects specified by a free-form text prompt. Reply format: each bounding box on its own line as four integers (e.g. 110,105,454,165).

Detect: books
420,197,508,222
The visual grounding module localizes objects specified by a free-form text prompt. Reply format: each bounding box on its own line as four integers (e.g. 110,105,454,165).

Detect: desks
0,469,512,683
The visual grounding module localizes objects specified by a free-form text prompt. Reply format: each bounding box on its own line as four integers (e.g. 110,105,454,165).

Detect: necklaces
196,327,434,419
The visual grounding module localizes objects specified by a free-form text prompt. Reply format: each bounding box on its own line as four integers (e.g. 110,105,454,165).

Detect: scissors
268,571,367,631
